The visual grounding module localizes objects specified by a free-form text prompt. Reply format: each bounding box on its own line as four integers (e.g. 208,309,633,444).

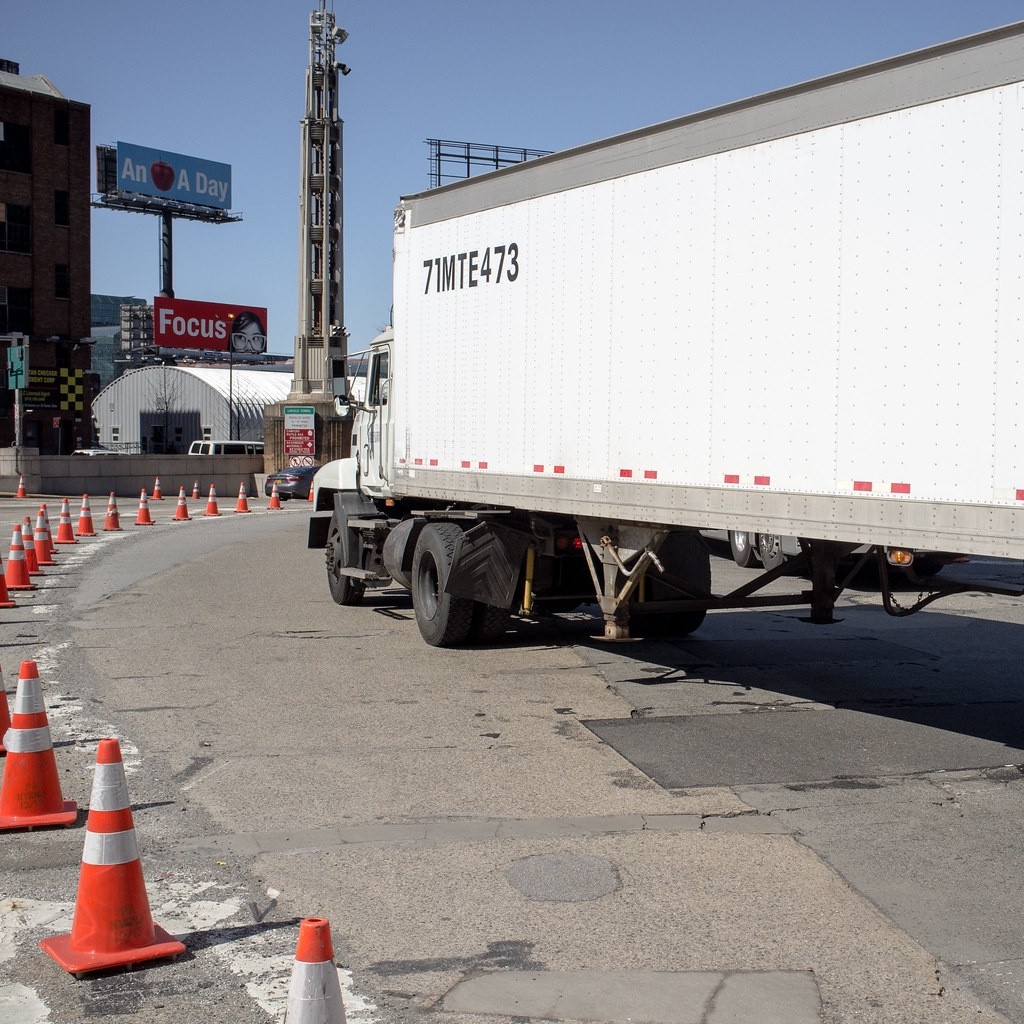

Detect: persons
227,311,267,354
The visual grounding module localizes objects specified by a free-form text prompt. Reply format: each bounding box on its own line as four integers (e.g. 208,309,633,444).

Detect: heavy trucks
306,21,1024,648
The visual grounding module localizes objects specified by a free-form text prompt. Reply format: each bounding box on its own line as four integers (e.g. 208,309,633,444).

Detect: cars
264,465,322,501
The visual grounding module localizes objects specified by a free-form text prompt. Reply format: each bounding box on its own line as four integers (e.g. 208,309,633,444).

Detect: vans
188,440,265,455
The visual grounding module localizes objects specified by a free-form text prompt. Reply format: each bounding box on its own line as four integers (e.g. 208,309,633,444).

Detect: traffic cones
74,493,98,536
267,480,283,510
40,503,59,555
16,474,28,499
34,511,57,565
202,483,222,517
170,484,193,521
151,475,166,500
5,524,38,591
191,480,200,499
232,481,251,513
53,497,79,544
103,491,123,531
0,663,12,754
0,550,17,608
283,915,348,1024
0,660,77,829
135,488,155,525
39,739,187,975
309,480,314,502
22,516,45,576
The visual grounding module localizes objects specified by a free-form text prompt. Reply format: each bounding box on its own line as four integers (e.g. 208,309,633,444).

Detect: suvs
70,449,131,456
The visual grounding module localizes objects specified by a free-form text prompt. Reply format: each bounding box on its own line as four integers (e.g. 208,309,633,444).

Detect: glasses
231,333,267,351
